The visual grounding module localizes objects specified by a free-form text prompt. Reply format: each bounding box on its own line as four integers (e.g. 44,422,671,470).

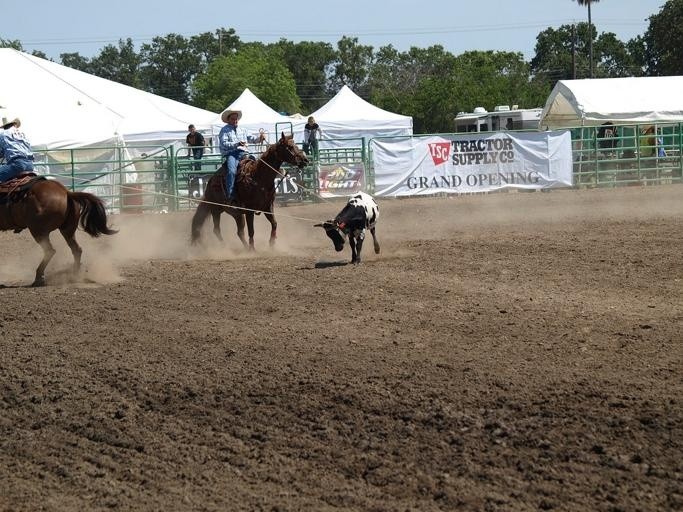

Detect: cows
313,190,380,266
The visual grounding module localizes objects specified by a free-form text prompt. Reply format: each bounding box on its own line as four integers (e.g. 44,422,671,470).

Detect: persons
303,116,323,155
186,124,206,170
0,117,35,183
639,125,661,185
572,135,585,150
219,111,265,205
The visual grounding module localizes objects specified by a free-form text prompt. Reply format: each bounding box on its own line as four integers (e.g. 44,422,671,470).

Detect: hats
221,109,242,123
0,116,21,129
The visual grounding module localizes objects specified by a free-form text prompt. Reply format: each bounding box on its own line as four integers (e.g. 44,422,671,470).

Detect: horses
0,175,120,287
190,131,310,253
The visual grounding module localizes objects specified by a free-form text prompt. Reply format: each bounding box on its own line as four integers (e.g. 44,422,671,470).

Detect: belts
10,156,33,160
